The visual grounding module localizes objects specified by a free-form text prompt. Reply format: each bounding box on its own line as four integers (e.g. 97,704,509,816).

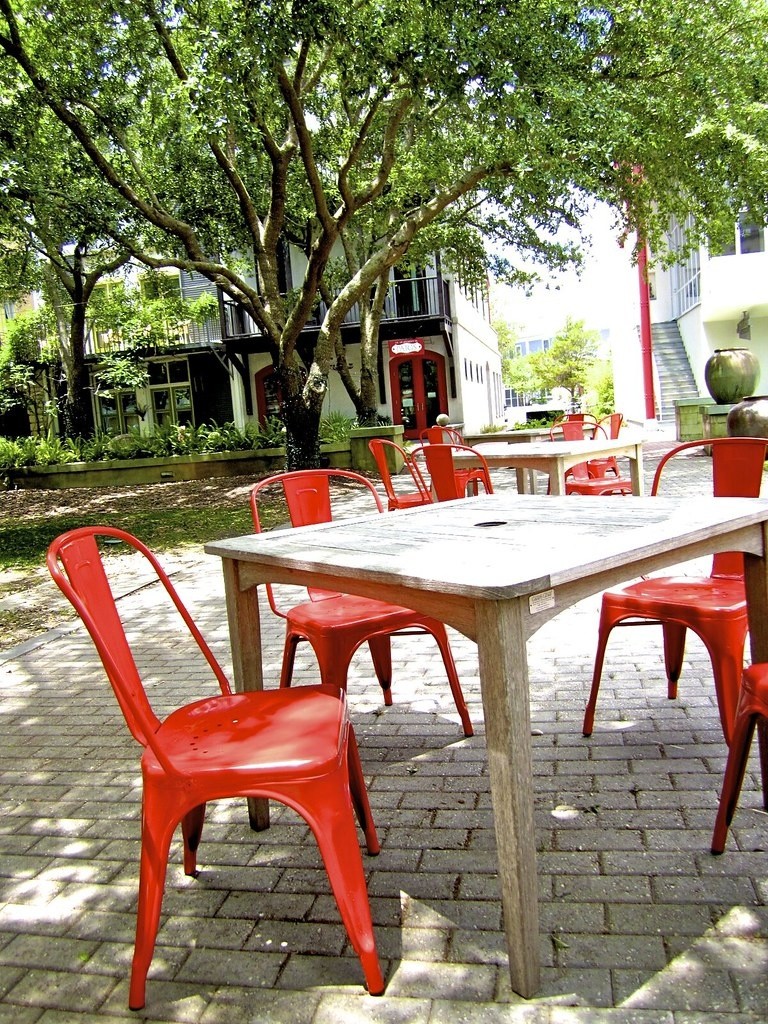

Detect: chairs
368,438,432,514
48,525,389,1016
246,468,475,742
554,414,599,425
710,663,768,856
412,442,495,502
550,421,634,497
564,412,626,497
420,428,492,500
579,437,768,754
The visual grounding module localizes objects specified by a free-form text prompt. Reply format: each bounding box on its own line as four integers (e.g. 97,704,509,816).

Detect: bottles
704,347,759,404
726,395,768,439
436,413,450,426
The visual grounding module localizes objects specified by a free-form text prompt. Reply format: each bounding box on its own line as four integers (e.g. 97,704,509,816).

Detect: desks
204,490,768,1001
431,440,648,498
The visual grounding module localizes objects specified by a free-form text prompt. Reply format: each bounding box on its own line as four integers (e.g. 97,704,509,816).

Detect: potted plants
464,410,597,444
